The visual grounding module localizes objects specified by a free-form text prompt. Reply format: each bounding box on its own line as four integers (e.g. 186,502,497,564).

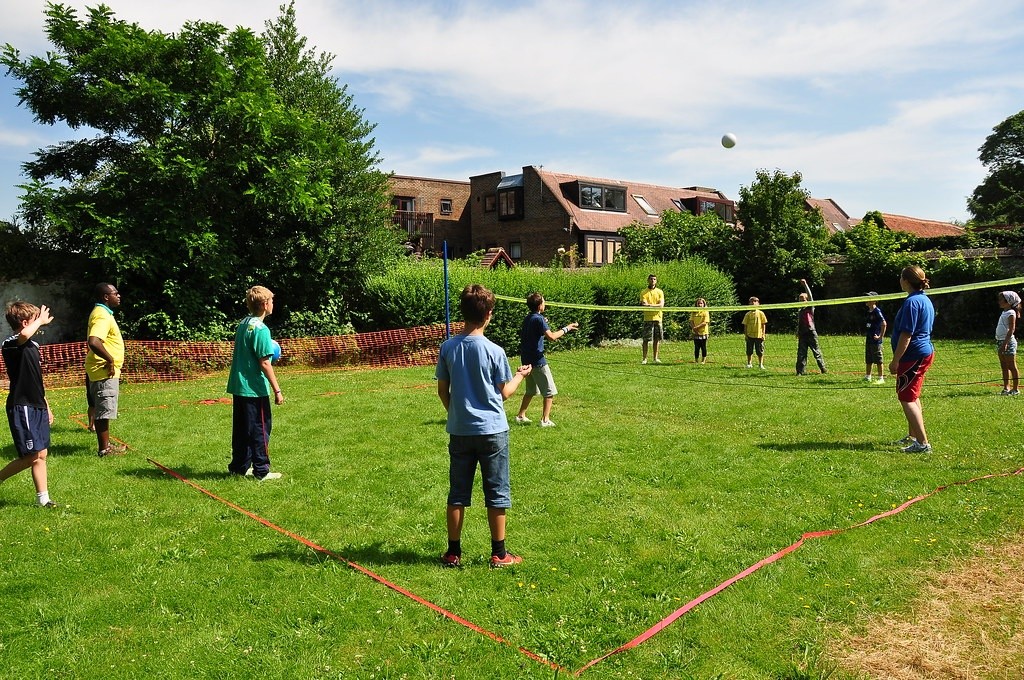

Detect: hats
866,292,878,302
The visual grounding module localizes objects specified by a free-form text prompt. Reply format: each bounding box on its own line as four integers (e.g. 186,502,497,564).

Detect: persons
639,275,665,364
742,297,767,369
863,291,887,385
84,282,127,456
515,293,579,427
995,290,1022,395
795,279,828,376
0,300,57,508
689,299,710,364
86,373,98,431
436,284,522,565
227,286,285,480
889,266,935,453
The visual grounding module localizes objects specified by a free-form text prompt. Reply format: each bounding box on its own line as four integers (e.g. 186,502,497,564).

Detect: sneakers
540,418,556,427
43,500,58,509
899,443,932,453
490,549,522,568
88,427,97,434
515,416,532,422
897,435,916,444
98,443,127,458
443,552,461,568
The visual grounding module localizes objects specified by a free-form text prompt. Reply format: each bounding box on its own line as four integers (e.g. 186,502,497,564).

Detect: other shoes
821,368,827,374
244,468,254,476
759,365,765,369
642,358,647,364
796,373,802,376
747,364,753,368
692,360,698,363
862,377,871,382
254,472,281,481
872,380,885,385
702,361,705,364
1001,389,1020,396
653,360,661,363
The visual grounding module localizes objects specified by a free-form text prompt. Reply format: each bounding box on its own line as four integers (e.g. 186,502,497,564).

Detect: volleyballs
722,133,736,148
270,339,282,365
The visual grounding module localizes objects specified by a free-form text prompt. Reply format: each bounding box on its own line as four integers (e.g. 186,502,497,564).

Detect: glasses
104,292,119,296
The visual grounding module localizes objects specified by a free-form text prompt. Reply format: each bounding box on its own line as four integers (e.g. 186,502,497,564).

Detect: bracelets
274,389,280,393
562,328,568,334
